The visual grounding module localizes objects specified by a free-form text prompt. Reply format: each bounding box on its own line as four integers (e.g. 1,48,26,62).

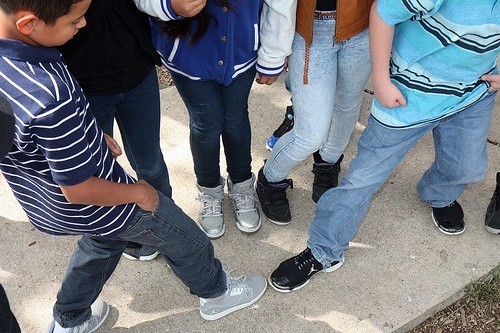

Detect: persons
0,0,267,333
56,0,174,261
134,0,297,237
256,0,500,293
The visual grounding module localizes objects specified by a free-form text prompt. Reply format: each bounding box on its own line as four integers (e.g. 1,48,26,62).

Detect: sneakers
265,106,296,151
312,151,344,205
431,200,466,235
47,299,109,333
195,176,226,238
200,275,267,320
122,241,160,260
255,159,293,225
268,247,344,293
485,172,500,234
227,172,261,234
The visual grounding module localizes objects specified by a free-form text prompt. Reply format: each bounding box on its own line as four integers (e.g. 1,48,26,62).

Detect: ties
315,12,336,16
